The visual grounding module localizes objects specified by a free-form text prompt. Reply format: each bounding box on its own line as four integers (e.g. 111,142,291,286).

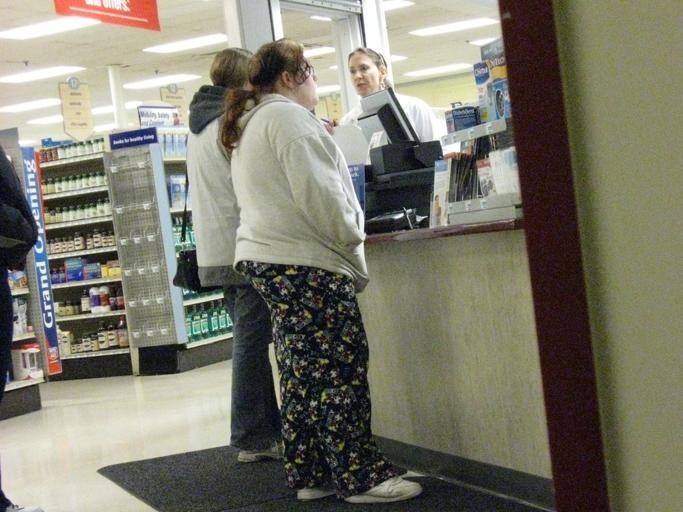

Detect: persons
180,46,291,464
322,45,442,169
217,34,426,504
0,138,42,512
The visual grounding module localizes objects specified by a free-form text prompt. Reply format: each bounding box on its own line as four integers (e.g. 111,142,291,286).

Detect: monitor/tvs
358,88,421,165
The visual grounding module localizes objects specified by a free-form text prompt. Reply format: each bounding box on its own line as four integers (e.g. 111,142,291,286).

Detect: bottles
54,316,129,353
180,286,223,299
40,170,107,194
45,227,116,255
53,283,125,317
36,136,104,163
48,257,121,284
183,299,234,343
42,196,112,226
172,215,196,253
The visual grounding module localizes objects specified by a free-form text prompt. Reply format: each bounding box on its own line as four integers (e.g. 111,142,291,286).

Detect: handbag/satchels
173,250,221,293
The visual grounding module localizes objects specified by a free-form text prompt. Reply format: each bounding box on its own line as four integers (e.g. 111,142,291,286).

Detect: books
432,151,480,228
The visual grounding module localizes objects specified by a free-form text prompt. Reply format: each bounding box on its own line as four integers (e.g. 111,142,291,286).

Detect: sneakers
238,449,284,462
297,488,337,500
344,476,422,503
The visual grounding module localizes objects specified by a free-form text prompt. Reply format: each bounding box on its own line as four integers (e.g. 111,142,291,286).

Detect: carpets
214,475,549,511
96,443,409,512
